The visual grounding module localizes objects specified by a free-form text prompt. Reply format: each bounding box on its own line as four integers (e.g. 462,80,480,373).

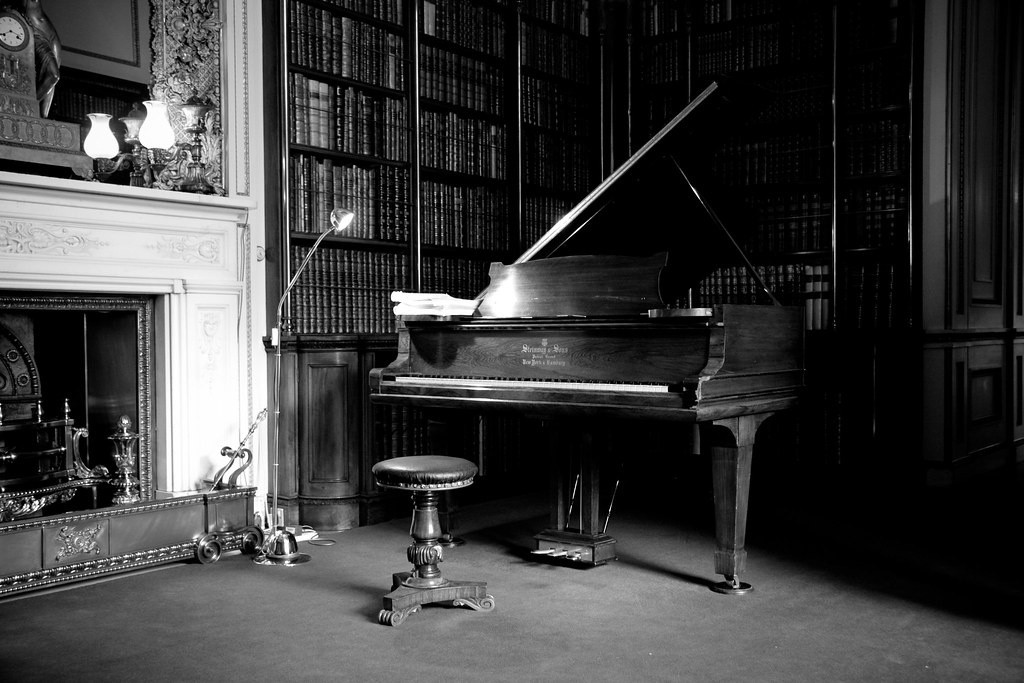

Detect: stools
371,456,495,627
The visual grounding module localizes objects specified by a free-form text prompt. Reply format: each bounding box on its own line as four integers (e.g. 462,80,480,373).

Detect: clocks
0,10,30,52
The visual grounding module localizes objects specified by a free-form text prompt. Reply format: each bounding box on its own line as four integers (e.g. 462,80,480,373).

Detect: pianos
367,248,802,598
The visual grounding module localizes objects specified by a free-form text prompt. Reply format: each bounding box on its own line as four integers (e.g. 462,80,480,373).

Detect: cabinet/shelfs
612,0,921,486
261,0,603,532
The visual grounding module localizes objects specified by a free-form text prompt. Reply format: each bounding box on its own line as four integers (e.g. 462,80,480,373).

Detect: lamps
82,98,213,194
253,206,357,568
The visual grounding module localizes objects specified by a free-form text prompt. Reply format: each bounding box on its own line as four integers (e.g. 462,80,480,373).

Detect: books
285,1,919,349
